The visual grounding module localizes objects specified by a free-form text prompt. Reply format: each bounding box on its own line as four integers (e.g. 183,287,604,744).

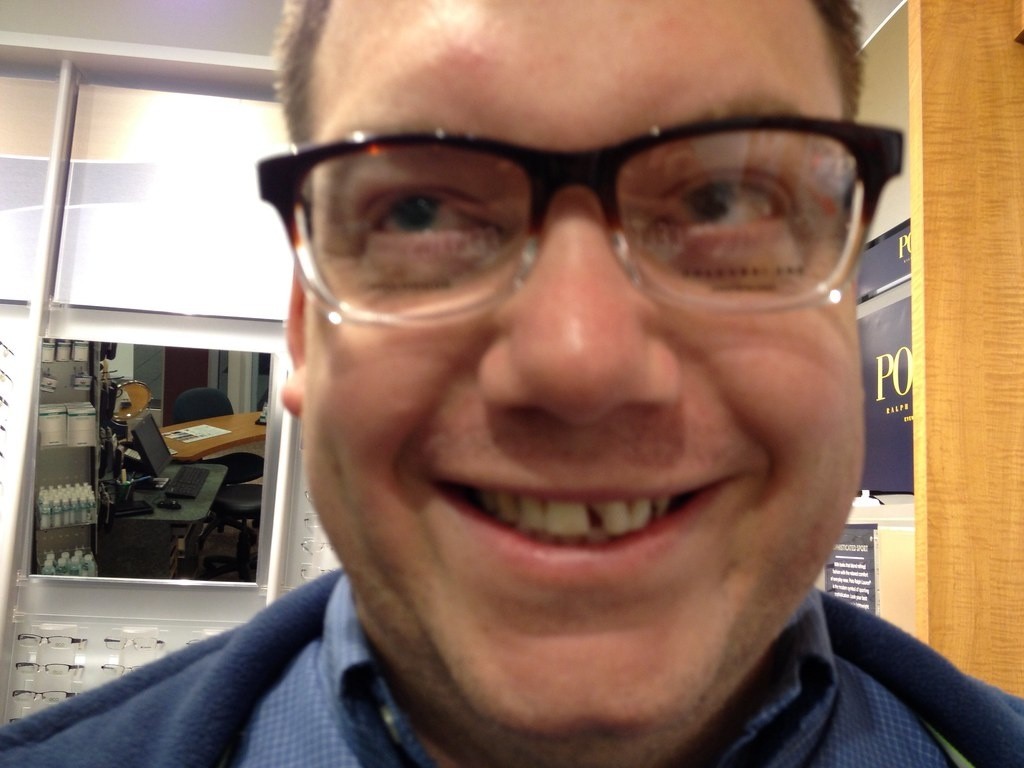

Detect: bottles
177,535,187,575
37,482,96,529
42,339,89,362
41,550,96,576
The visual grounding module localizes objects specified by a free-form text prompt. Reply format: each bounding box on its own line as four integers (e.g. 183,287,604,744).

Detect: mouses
158,501,183,510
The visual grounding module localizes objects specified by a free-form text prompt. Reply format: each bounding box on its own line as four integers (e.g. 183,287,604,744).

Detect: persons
0,0,1024,768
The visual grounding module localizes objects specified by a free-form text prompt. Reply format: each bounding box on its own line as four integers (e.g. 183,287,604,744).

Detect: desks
159,410,267,460
96,464,228,578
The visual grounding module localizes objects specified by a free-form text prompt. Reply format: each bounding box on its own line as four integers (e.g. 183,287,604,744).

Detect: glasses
258,110,910,326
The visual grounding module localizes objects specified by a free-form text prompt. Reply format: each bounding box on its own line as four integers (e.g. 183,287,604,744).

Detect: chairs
169,387,265,549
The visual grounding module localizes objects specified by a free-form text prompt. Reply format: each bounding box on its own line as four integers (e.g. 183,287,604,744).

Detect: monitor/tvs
131,414,173,489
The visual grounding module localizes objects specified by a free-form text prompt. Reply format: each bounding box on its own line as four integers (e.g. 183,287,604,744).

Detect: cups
111,483,133,505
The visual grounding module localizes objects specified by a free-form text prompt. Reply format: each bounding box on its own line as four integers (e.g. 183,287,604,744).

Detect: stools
196,483,262,582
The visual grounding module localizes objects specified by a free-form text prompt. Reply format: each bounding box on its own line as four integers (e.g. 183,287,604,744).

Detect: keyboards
166,466,210,499
